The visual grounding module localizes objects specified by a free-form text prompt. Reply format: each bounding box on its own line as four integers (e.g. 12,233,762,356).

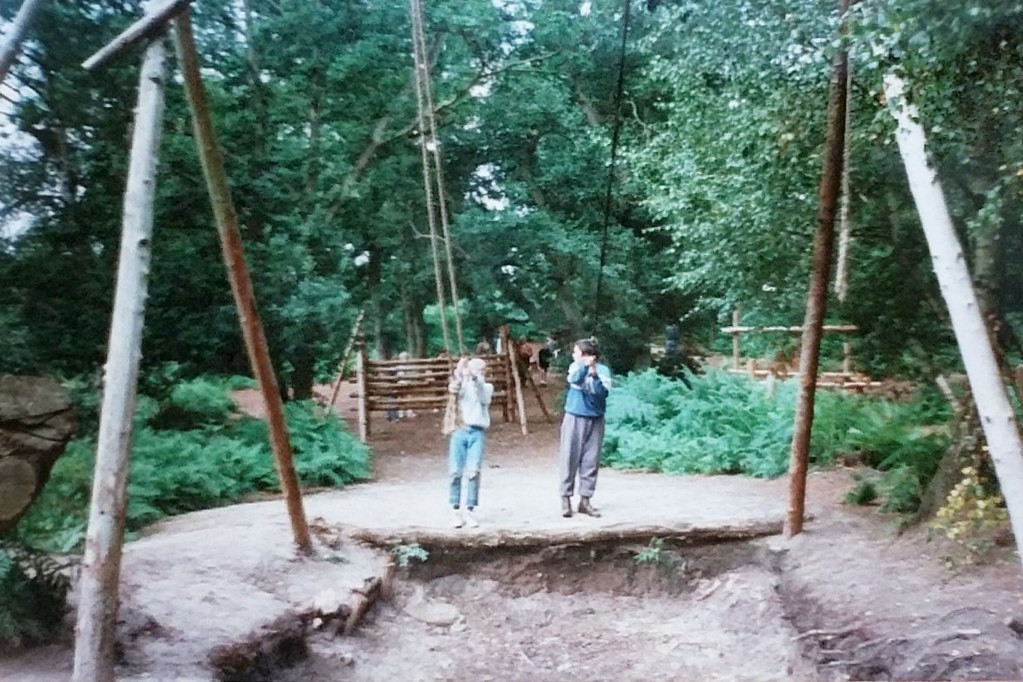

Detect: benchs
349,325,515,447
725,346,882,396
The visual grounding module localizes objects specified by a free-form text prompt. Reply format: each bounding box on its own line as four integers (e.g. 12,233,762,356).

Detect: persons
558,340,610,518
476,343,503,392
448,356,493,528
384,351,450,420
518,336,552,387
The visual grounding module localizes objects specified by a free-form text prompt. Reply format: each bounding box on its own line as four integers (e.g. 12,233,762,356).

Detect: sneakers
562,496,574,518
466,511,478,527
452,508,466,527
578,497,602,518
